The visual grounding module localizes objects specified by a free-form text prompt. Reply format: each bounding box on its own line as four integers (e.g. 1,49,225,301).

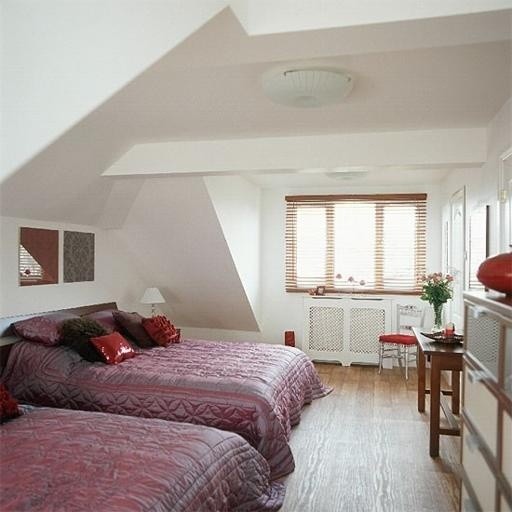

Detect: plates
420,330,465,344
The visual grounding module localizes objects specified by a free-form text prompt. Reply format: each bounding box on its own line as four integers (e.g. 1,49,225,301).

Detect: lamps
260,64,356,109
140,287,167,315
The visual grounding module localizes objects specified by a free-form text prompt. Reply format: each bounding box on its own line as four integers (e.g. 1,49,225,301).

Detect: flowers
417,272,456,328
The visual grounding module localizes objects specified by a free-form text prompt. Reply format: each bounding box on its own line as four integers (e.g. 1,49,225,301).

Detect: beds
0,300,332,485
0,400,282,512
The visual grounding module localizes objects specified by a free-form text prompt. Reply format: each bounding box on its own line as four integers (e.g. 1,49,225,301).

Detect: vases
431,303,445,334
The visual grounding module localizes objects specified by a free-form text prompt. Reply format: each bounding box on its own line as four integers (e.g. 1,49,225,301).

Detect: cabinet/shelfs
458,285,512,510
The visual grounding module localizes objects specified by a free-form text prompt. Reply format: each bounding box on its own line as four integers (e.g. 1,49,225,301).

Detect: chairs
377,303,426,381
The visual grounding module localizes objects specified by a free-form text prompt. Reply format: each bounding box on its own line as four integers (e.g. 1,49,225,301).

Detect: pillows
1,385,24,423
11,309,181,363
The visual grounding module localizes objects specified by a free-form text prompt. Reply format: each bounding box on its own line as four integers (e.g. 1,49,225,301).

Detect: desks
410,326,464,457
